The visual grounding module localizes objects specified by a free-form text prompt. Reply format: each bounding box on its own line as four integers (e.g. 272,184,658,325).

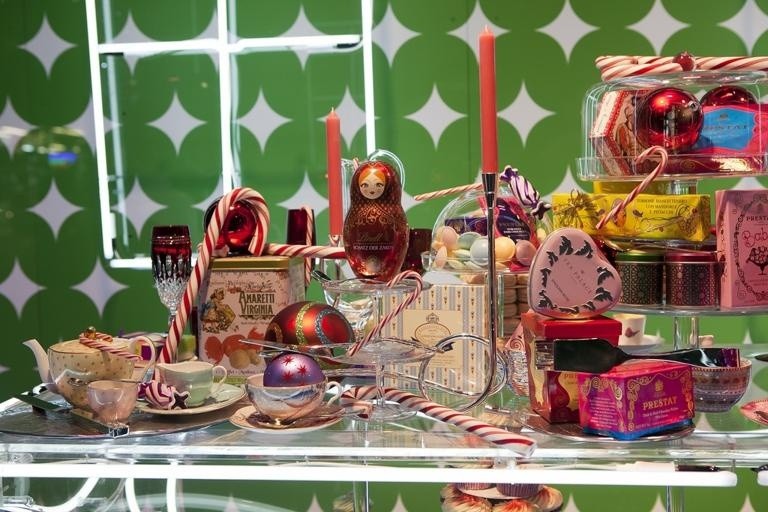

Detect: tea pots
23,326,157,408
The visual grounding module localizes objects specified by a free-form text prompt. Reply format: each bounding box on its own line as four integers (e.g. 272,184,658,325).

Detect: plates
135,382,248,414
740,400,768,430
617,335,666,351
230,405,347,436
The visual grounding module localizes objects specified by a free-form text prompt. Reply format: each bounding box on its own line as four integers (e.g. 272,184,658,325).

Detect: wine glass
151,224,193,327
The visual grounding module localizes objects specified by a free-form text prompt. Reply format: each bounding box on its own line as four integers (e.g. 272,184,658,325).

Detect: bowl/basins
691,358,752,414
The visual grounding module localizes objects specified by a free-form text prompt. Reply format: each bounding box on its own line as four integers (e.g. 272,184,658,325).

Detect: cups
612,315,646,344
87,381,139,423
674,315,700,352
243,373,344,424
158,362,227,409
